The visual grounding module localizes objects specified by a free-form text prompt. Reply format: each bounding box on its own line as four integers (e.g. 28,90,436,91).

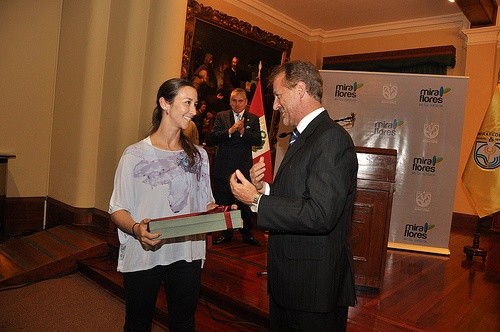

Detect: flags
247,75,274,185
460,85,500,219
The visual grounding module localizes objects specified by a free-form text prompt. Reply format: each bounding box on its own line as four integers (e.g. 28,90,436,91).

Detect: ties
236,113,240,120
288,128,300,149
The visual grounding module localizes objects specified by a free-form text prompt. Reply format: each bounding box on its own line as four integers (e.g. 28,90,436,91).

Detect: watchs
249,192,262,213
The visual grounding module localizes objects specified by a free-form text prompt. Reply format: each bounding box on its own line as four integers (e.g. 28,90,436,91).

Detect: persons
228,61,357,332
187,39,273,145
107,77,240,332
208,88,263,247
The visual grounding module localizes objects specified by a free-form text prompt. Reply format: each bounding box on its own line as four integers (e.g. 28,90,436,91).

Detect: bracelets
132,223,139,237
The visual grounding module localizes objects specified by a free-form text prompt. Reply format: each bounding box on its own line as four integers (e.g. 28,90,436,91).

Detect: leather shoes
242,236,260,246
212,235,234,245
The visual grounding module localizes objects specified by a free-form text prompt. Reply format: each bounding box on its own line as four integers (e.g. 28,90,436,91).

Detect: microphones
278,132,292,138
